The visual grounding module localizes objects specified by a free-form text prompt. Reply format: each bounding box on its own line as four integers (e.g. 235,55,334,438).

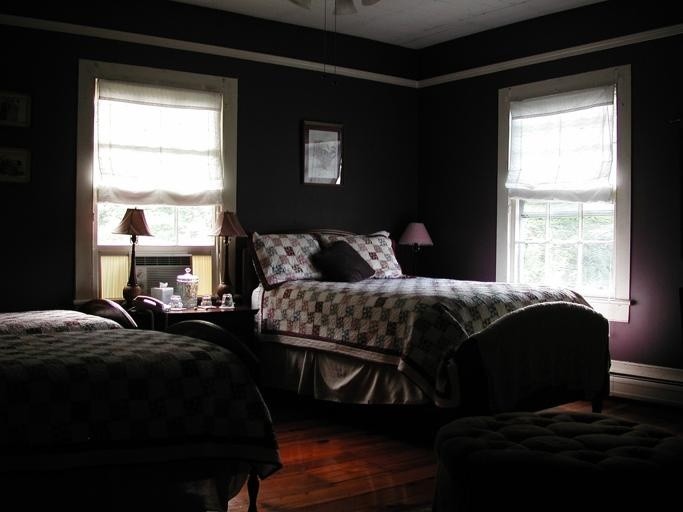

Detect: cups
170,295,182,311
201,295,212,307
221,293,233,307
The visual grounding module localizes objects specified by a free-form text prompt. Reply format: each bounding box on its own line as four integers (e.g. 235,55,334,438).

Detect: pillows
247,229,405,292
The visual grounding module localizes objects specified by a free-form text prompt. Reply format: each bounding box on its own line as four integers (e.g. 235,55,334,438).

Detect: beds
0,310,285,510
250,226,611,449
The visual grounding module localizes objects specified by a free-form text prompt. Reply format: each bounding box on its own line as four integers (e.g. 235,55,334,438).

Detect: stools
431,409,682,511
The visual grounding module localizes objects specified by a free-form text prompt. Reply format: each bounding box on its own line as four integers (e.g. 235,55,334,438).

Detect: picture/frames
300,119,345,189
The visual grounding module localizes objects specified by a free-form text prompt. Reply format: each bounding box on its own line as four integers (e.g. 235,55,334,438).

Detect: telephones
132,295,172,314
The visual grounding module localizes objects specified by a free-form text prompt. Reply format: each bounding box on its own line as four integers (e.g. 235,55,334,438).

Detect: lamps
116,208,151,307
208,209,249,306
396,222,435,278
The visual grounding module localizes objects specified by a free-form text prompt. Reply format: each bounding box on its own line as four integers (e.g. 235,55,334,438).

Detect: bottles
175,268,198,308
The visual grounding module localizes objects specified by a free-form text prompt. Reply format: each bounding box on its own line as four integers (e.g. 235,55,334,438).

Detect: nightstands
130,305,259,362
